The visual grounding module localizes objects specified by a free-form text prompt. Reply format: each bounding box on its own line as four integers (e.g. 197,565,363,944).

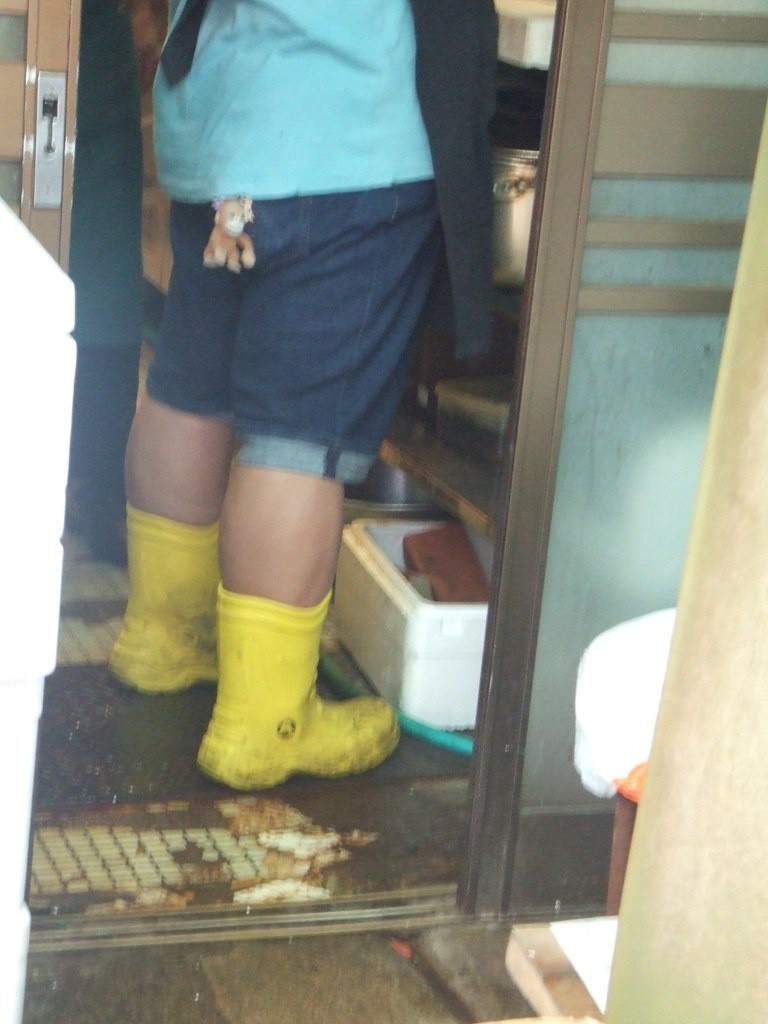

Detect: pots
492,148,546,287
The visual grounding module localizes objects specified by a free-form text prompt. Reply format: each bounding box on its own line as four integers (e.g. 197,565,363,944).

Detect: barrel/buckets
343,458,440,523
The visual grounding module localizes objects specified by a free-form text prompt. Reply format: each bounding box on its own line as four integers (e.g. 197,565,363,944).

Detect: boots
195,579,398,789
109,503,221,694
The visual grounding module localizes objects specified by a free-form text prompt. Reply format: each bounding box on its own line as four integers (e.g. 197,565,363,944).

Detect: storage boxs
334,517,488,732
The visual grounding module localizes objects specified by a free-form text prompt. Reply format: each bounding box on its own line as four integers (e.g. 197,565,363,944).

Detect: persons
68,1,167,556
112,0,496,791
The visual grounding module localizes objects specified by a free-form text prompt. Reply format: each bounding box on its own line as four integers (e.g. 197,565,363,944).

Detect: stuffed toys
203,201,256,273
